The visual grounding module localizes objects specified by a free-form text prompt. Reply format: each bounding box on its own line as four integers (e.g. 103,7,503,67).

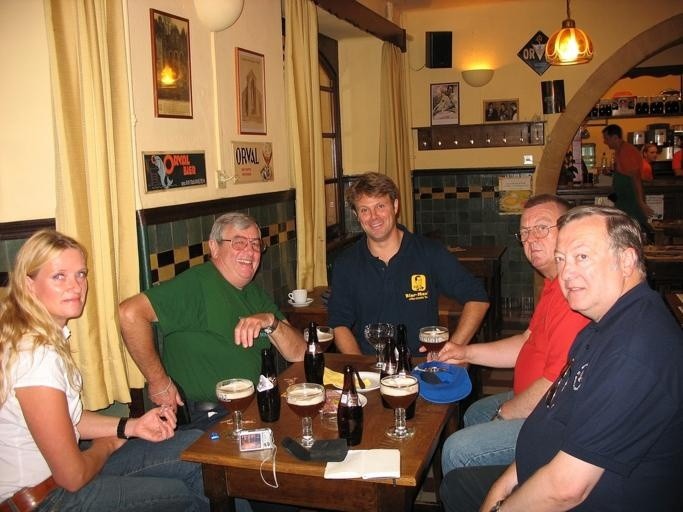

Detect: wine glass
377,374,419,442
363,323,396,369
417,325,449,362
214,377,256,441
283,382,327,451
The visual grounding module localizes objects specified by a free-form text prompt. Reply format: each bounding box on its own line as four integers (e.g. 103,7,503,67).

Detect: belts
1,476,59,511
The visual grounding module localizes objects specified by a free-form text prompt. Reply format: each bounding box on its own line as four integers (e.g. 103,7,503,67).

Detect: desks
642,243,681,295
661,286,682,327
179,349,474,510
648,217,682,239
274,281,470,343
436,239,508,341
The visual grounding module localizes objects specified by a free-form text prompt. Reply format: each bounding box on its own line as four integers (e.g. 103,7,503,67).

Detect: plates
286,298,313,308
318,389,368,414
332,371,380,393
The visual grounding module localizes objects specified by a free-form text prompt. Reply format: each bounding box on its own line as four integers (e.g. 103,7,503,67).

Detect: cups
287,289,307,303
302,325,334,352
319,391,341,432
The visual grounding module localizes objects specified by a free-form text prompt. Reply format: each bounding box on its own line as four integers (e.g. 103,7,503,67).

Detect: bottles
336,364,364,448
256,348,281,423
301,320,325,384
653,228,664,251
600,151,614,168
379,324,416,421
589,95,683,117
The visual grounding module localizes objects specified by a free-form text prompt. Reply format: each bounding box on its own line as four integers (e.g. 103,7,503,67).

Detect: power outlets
211,168,229,191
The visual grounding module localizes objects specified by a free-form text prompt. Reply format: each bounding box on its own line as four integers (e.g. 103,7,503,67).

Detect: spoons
420,128,539,147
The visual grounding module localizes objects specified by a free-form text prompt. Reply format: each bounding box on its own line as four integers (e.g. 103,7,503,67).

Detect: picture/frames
427,80,460,125
233,46,267,136
480,96,520,124
147,7,194,122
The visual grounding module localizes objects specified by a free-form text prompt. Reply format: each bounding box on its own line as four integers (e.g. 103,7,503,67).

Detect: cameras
238,427,273,452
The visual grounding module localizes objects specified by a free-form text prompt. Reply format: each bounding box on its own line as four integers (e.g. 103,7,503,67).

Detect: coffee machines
626,131,647,153
647,123,673,167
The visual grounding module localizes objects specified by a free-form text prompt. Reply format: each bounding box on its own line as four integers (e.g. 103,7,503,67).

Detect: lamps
544,1,594,68
460,65,494,89
188,0,245,38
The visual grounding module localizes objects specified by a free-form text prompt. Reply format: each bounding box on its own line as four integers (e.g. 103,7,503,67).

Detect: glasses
545,359,574,409
514,223,556,242
220,235,268,254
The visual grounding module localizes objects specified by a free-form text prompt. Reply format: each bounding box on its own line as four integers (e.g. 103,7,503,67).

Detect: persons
601,124,654,245
638,141,655,180
444,85,457,112
435,203,682,512
438,189,598,478
1,228,261,512
326,171,495,356
510,103,518,120
114,208,311,431
486,103,496,121
671,149,682,178
112,413,131,441
497,103,510,121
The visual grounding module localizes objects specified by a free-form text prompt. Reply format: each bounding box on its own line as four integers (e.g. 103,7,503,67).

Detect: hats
411,360,472,404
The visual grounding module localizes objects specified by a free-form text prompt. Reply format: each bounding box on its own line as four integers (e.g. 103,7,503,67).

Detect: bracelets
489,500,503,512
148,377,172,398
494,404,505,420
257,315,279,332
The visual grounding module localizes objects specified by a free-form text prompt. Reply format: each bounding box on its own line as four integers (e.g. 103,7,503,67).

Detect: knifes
352,367,364,390
